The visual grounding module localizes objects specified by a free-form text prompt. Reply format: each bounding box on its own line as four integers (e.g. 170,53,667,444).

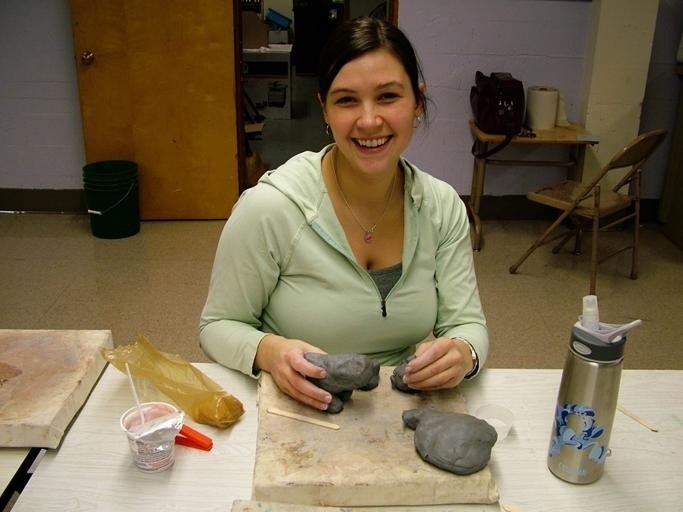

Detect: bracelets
454,338,478,378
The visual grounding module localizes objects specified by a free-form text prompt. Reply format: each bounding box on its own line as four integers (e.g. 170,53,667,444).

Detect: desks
467,119,600,252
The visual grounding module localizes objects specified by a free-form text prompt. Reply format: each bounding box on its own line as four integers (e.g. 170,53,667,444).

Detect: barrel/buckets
82,161,141,239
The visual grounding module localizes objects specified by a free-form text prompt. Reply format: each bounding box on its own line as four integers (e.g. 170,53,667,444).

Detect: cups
120,401,180,473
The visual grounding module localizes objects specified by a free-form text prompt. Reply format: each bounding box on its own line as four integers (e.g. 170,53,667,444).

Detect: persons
196,16,489,412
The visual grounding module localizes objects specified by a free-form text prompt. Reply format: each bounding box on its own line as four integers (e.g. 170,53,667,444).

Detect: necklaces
329,144,397,244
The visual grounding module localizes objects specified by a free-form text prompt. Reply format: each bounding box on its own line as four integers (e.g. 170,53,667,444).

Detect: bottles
548,293,642,484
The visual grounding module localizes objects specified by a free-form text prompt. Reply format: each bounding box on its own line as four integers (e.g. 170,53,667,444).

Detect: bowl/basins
268,44,293,52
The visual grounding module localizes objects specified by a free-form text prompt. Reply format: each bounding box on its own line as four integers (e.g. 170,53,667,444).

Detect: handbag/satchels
470,70,523,135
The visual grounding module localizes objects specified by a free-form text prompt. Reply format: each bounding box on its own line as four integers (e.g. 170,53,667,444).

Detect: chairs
508,126,669,293
